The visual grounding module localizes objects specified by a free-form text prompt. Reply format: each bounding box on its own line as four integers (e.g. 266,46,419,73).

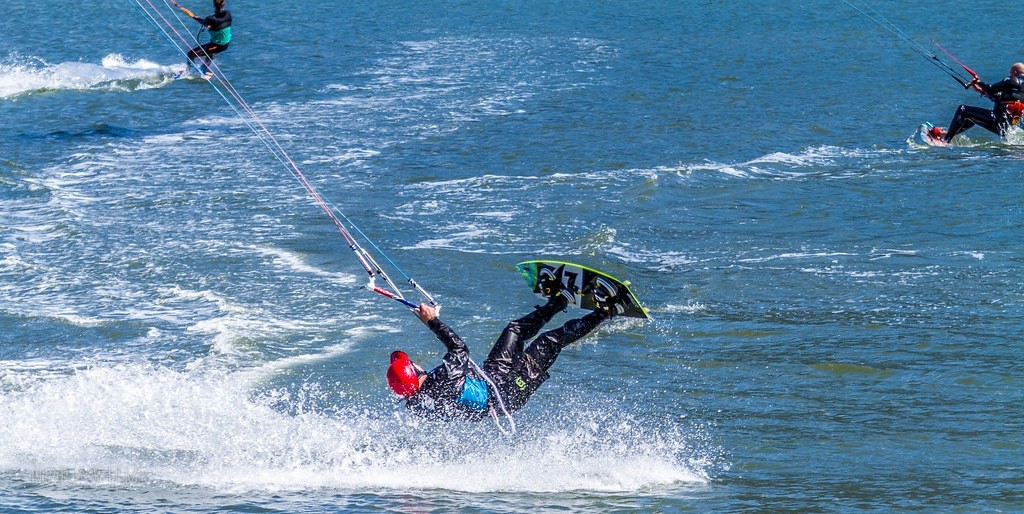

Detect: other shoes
199,66,207,74
932,127,947,144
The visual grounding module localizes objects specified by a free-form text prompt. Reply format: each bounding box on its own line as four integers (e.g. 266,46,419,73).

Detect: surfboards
172,66,212,81
922,124,947,147
516,258,650,320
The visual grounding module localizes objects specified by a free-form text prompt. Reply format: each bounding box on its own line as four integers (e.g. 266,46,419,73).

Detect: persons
387,268,625,422
931,63,1024,144
187,0,232,75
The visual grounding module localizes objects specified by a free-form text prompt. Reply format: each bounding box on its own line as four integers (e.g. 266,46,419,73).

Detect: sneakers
538,277,568,306
594,288,617,319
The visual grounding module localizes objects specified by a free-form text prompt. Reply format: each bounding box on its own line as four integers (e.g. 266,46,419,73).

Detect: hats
387,350,419,397
